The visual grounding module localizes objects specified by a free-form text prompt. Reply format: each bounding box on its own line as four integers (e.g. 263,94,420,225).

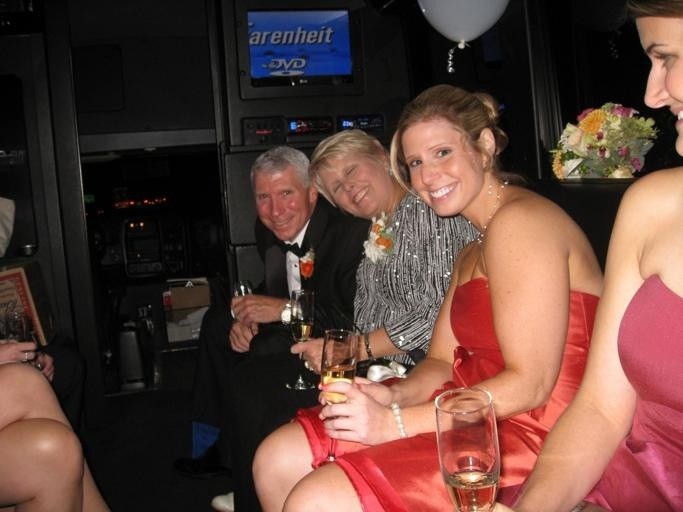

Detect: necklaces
476,180,509,244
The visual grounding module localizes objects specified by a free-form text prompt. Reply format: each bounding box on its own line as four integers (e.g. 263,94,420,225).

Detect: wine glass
434,388,499,512
4,305,46,371
283,291,315,391
232,279,252,298
310,329,358,468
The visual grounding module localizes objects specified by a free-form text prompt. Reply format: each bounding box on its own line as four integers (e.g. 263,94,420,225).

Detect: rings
25,352,28,360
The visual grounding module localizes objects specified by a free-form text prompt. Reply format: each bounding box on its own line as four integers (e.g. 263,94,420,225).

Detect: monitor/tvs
234,0,366,101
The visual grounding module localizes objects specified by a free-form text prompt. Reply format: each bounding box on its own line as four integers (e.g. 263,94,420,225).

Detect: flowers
548,101,662,180
363,209,394,264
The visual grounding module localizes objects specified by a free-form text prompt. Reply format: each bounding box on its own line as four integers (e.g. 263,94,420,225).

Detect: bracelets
363,333,373,361
391,403,406,438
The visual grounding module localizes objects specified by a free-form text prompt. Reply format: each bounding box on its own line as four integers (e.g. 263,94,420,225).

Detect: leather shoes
171,443,232,479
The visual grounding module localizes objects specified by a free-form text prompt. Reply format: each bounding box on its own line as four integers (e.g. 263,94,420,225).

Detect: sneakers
209,490,236,511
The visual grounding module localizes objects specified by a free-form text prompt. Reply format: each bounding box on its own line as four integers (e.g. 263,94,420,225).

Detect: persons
177,146,374,479
253,83,602,512
211,127,482,512
1,363,111,512
1,339,54,381
490,0,683,512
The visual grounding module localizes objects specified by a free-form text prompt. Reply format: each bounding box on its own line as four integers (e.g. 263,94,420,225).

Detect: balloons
419,0,510,50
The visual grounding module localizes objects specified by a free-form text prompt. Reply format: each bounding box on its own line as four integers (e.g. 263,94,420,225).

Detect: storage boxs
168,281,212,309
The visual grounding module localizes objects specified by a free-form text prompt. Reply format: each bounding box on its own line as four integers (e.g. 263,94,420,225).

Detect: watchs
280,300,293,325
